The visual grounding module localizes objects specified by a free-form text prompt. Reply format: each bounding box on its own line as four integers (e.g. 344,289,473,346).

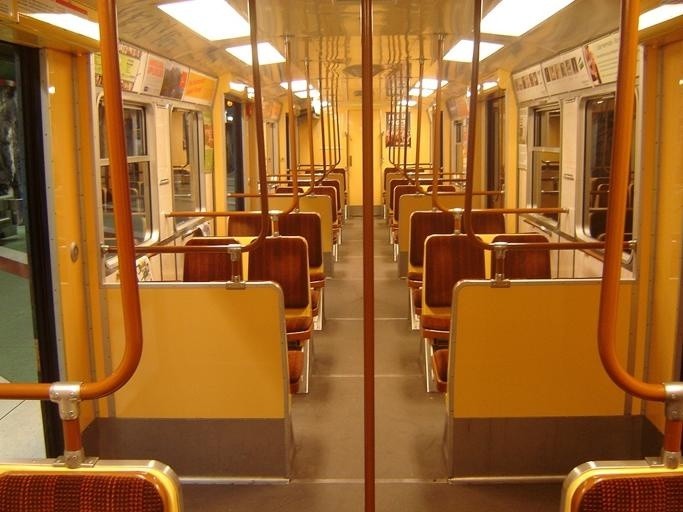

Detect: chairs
222,211,329,334
170,234,314,397
557,452,683,512
0,454,186,512
409,209,508,330
380,166,457,263
0,166,350,511
422,231,561,397
272,165,349,263
